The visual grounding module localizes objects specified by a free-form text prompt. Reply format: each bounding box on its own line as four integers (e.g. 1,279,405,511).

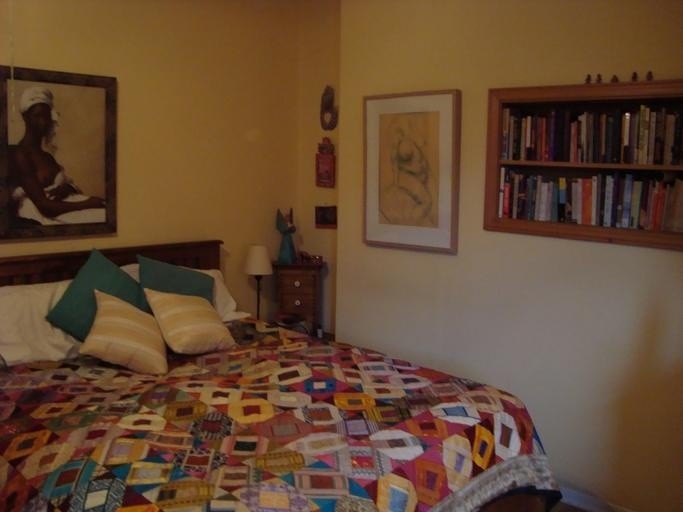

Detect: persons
10,86,107,226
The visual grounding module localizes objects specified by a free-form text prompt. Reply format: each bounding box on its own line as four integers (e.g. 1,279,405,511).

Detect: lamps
246,243,274,323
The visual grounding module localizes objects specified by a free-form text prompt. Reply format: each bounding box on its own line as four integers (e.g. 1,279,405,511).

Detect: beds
0,239,562,512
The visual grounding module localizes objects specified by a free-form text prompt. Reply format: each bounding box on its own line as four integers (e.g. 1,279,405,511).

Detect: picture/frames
315,205,337,230
315,138,337,189
361,88,463,257
319,84,337,133
1,63,118,242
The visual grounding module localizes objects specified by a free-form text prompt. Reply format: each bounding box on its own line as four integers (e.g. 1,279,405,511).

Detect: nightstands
277,263,323,329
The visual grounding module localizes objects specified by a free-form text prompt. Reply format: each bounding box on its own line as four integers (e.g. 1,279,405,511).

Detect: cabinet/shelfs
482,82,683,251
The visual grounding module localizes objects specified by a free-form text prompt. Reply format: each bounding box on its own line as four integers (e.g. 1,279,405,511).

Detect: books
500,105,682,165
498,167,683,234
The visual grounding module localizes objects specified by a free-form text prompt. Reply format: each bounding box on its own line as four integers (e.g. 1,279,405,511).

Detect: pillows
45,249,143,341
145,288,236,353
140,254,212,307
1,263,252,365
77,290,167,377
